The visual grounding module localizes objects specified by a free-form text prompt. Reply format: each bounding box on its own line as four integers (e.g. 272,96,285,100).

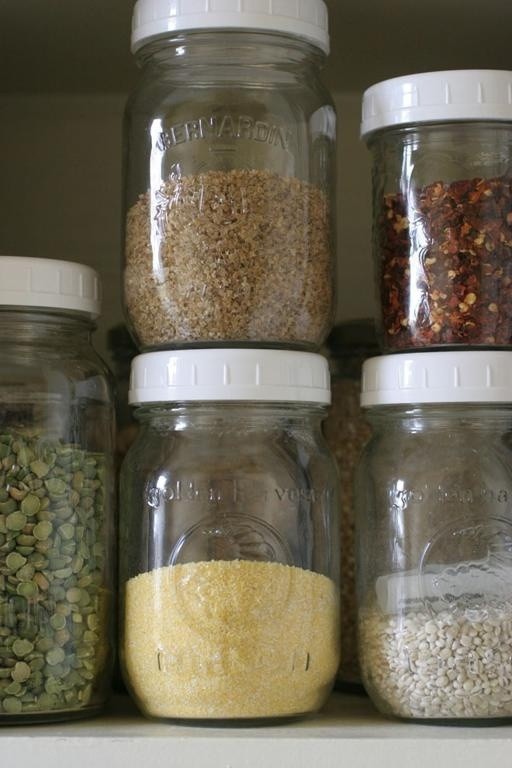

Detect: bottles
123,1,336,347
331,318,382,695
357,70,511,350
355,352,512,726
118,346,344,730
100,324,145,698
1,255,118,719
